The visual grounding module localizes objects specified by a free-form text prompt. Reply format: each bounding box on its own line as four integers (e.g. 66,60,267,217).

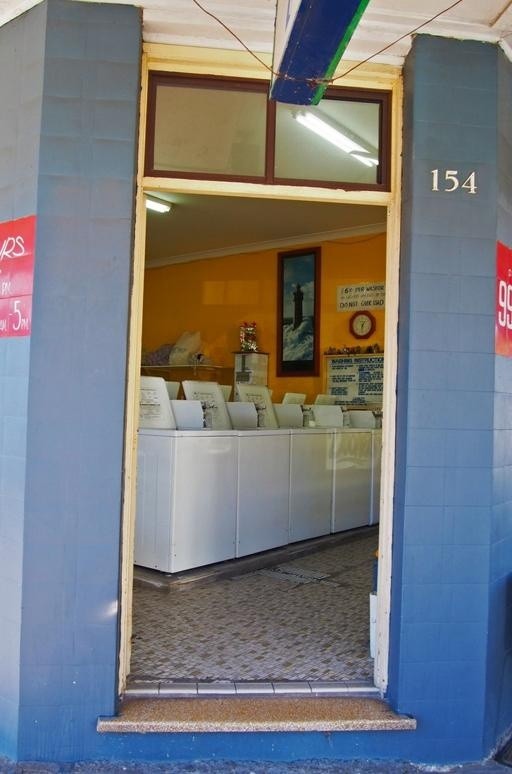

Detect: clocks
350,311,376,339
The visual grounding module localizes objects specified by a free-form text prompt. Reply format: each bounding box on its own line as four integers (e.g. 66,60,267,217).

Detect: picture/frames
322,353,384,410
277,246,320,377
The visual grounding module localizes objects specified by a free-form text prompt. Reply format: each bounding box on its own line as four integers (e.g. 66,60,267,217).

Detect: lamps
143,193,172,213
291,106,379,167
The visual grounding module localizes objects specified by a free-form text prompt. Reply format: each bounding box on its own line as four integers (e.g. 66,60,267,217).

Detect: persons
292,281,304,327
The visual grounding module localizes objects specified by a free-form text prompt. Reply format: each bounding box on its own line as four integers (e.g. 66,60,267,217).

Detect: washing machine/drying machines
137,375,382,574
